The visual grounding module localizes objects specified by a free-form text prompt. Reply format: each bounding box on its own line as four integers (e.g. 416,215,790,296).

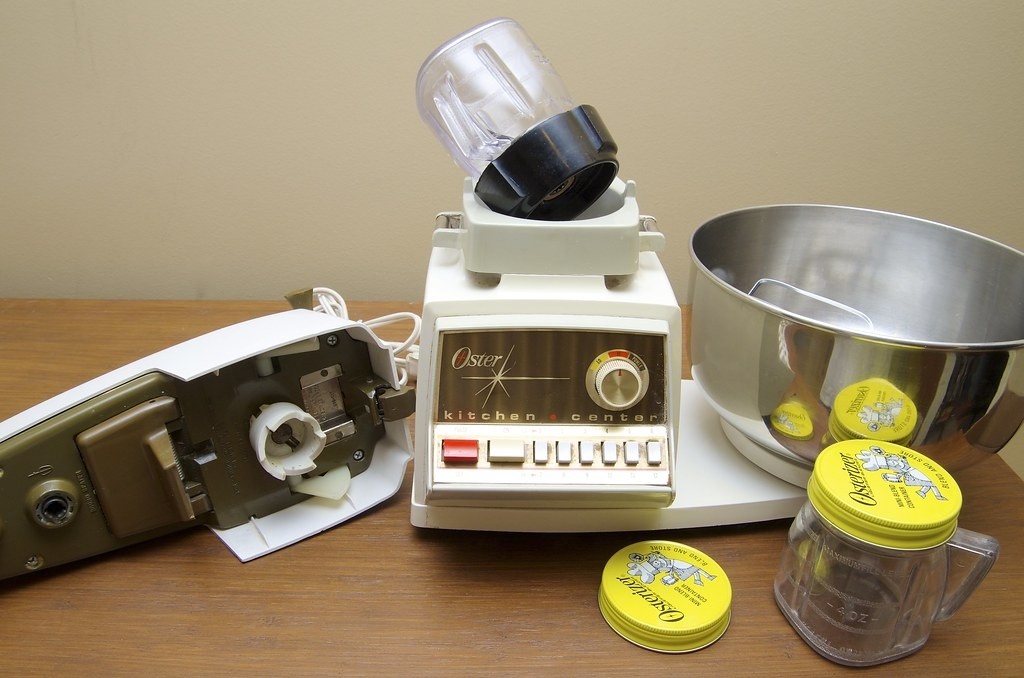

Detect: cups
773,438,1000,671
816,374,919,452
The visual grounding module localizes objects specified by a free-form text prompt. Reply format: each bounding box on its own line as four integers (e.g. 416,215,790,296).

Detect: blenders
0,13,814,588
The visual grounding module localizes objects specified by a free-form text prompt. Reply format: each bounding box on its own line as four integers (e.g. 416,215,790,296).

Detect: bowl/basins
688,204,1024,473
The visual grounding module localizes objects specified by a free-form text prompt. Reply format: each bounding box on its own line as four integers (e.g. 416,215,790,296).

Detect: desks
0,294,1024,678
